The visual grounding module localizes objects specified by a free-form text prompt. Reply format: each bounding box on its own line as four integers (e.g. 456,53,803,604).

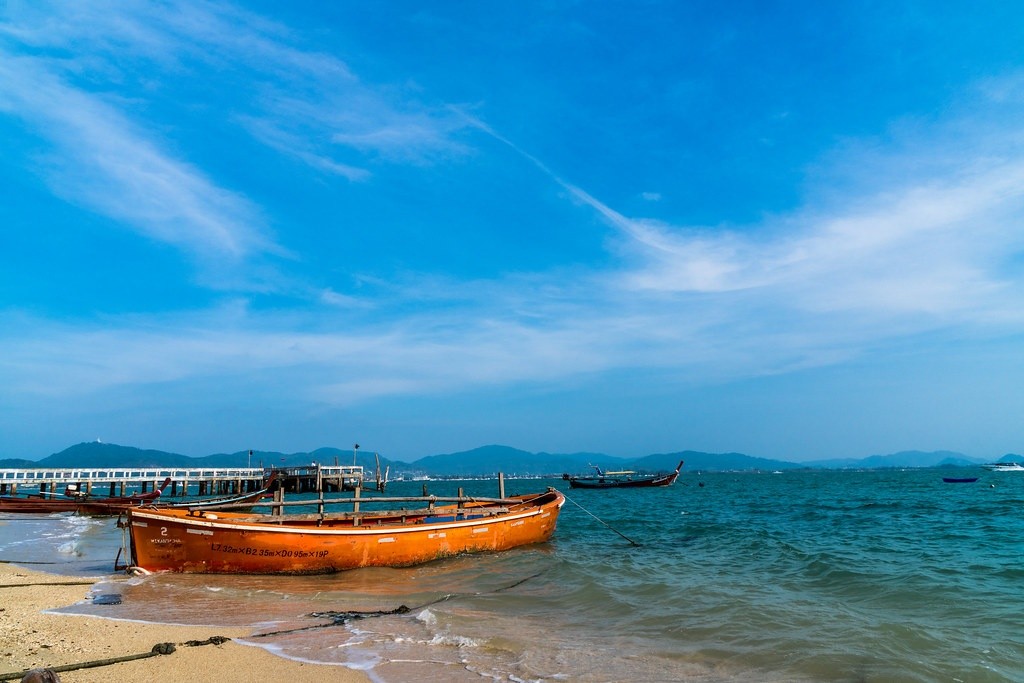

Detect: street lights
248,448,253,467
354,442,359,471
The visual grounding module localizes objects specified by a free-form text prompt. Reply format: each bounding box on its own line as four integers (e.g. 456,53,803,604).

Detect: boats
0,477,171,514
107,471,276,519
943,477,978,482
989,462,1024,472
113,473,565,575
563,461,684,488
